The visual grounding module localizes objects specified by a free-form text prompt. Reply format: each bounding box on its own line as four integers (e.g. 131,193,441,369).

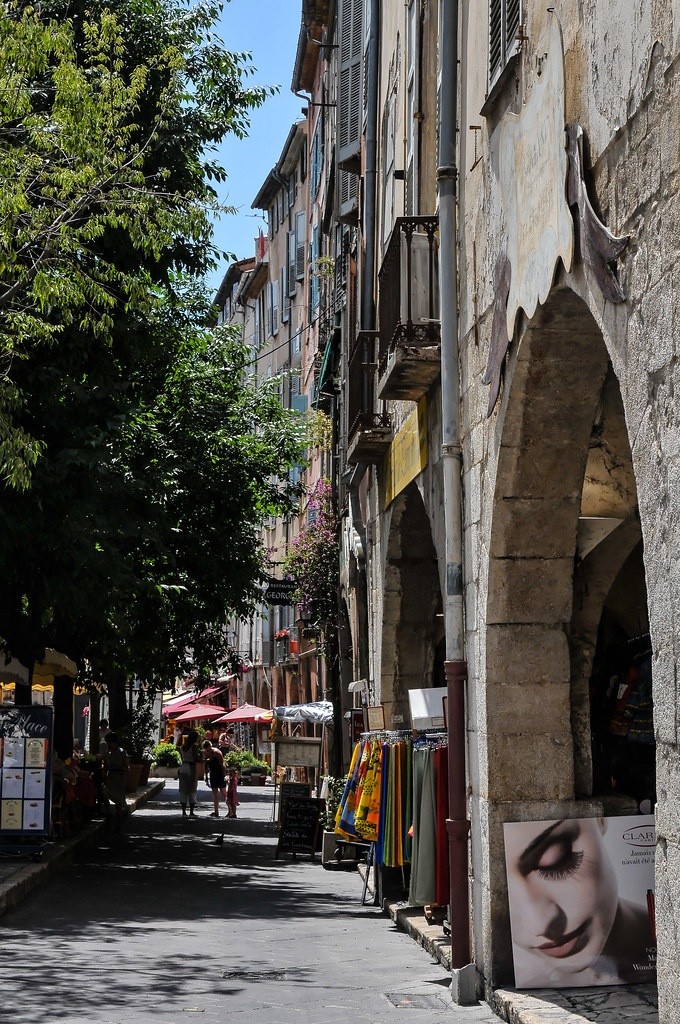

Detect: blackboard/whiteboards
280,781,311,799
276,796,327,854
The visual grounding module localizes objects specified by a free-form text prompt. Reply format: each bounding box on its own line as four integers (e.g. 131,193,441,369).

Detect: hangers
359,727,449,753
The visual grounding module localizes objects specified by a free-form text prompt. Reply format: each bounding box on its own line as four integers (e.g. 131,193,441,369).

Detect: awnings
162,684,229,716
0,642,92,702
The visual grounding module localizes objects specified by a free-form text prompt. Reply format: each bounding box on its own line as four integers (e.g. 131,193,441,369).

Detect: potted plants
122,712,152,796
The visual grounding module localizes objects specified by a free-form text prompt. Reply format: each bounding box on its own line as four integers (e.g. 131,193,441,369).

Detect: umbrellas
168,704,228,731
211,701,273,762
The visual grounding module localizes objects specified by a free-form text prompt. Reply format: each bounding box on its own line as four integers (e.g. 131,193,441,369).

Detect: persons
201,740,227,817
503,817,650,990
225,769,241,818
99,718,112,743
179,731,206,820
219,728,239,756
104,732,128,813
